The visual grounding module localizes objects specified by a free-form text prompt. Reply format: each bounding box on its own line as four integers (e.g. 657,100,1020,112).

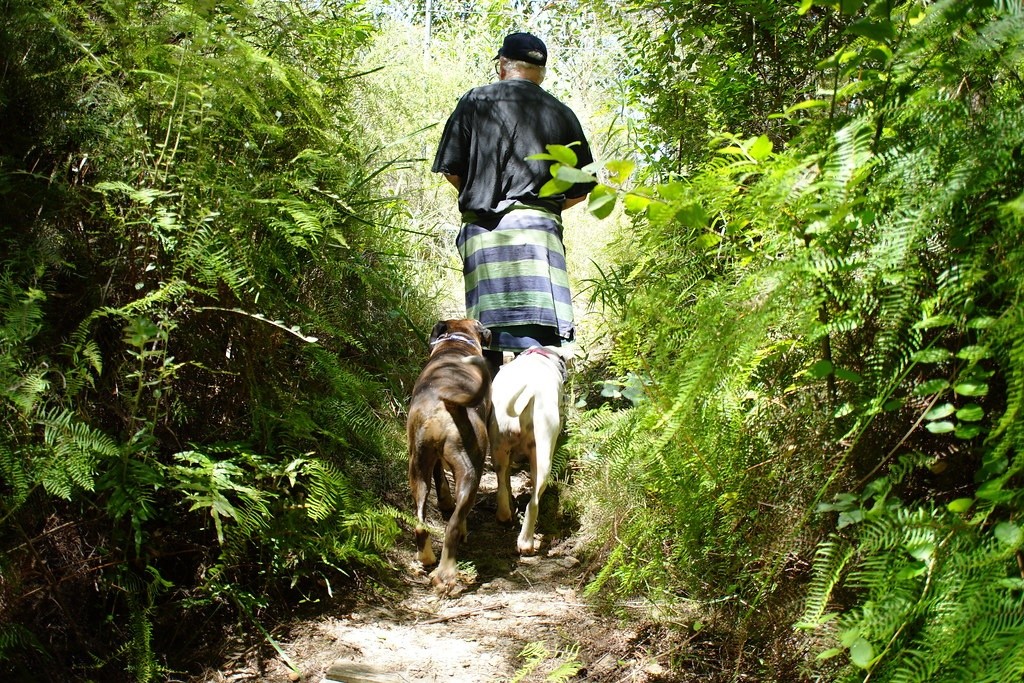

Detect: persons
431,32,594,487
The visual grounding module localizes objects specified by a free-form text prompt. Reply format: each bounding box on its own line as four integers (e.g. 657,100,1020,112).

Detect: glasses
495,61,501,73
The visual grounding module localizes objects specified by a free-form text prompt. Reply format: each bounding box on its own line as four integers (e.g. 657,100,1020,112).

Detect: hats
492,32,547,66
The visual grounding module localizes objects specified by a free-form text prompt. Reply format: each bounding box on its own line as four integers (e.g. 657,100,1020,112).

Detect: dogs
489,333,577,555
407,318,492,599
